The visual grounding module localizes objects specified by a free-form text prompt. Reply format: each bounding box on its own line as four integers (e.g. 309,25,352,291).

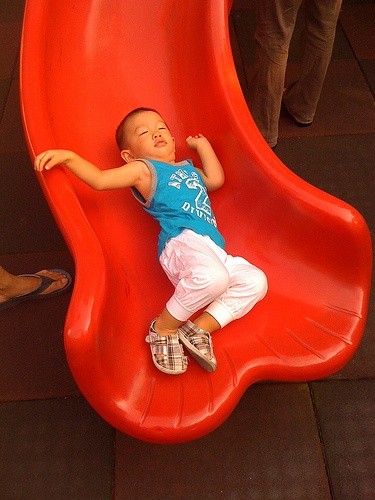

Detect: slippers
283,87,314,125
0,268,72,311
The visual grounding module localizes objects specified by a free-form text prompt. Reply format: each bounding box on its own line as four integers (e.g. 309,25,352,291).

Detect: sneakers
178,320,218,372
145,319,188,374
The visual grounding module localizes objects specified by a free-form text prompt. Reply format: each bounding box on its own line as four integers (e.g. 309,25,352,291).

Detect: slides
19,1,372,445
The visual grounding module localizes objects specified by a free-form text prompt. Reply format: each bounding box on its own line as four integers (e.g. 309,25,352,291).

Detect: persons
250,0,343,150
0,263,72,312
34,107,268,375
